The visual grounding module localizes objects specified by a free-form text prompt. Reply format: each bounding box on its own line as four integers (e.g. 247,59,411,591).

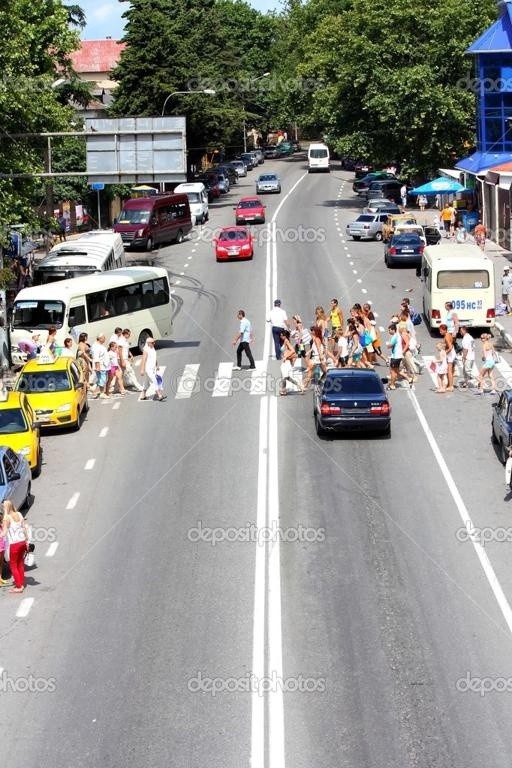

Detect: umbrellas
410,175,471,213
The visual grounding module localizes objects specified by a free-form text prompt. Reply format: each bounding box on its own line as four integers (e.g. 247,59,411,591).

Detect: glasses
148,341,155,345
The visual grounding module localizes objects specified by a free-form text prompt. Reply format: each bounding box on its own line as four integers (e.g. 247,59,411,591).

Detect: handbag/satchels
347,341,364,356
88,369,99,386
439,226,444,230
452,342,464,355
495,302,507,316
358,330,374,347
410,314,424,326
492,352,503,364
23,550,36,568
283,339,297,360
155,375,165,386
292,324,313,348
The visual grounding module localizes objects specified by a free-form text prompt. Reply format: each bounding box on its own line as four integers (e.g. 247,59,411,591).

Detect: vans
173,182,210,225
113,193,194,253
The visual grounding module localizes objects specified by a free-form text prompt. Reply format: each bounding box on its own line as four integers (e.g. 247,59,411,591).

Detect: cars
312,367,391,437
1,345,90,520
196,139,301,224
213,227,257,262
339,147,442,269
489,388,511,468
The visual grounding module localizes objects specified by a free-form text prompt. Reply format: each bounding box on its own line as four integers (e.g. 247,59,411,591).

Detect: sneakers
0,578,26,594
506,311,512,316
503,487,512,501
232,366,242,372
245,366,258,372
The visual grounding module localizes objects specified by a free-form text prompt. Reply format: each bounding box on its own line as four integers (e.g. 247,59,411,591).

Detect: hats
274,299,282,307
293,313,302,323
504,265,510,271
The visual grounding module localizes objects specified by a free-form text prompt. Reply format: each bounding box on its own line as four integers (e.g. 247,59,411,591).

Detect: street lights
243,71,272,153
158,87,215,196
42,75,70,99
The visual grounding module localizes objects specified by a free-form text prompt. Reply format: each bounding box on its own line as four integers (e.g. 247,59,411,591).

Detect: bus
32,229,126,286
8,264,173,368
421,242,495,334
307,144,331,172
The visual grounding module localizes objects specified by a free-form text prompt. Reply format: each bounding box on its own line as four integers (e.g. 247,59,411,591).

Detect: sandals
87,386,168,402
277,360,500,397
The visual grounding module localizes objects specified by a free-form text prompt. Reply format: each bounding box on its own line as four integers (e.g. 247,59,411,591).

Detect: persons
500,266,511,315
56,212,67,241
438,202,487,251
0,512,9,585
1,498,30,593
400,183,409,210
416,192,428,211
15,299,170,403
231,310,256,371
266,292,502,396
